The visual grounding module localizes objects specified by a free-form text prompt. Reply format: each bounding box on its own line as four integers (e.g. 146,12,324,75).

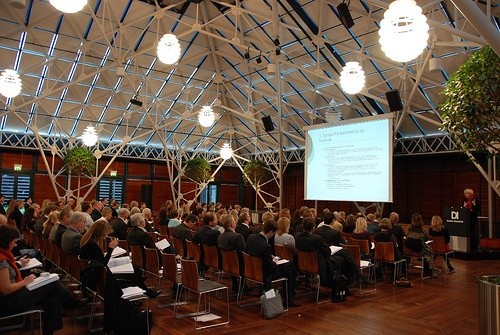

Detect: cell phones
18,253,31,260
274,255,281,260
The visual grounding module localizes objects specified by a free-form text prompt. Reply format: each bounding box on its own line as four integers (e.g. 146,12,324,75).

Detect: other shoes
447,270,455,276
151,290,163,298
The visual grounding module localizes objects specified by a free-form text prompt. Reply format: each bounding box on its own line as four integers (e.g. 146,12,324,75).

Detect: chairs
0,219,455,335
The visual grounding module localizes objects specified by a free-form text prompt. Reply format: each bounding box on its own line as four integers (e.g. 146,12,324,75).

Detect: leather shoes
358,279,376,286
286,302,301,307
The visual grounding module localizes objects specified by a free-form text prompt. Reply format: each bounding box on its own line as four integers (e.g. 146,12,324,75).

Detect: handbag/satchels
260,290,284,318
331,274,348,302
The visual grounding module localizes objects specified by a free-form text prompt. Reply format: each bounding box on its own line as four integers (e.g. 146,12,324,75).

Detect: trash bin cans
478,274,500,335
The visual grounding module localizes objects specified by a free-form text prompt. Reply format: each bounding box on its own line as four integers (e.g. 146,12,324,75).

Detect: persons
313,213,346,247
252,206,382,250
374,218,404,282
0,224,88,335
406,213,433,276
245,218,302,307
388,212,407,273
161,198,251,237
0,195,81,257
274,217,302,289
352,217,384,282
461,188,481,236
217,214,252,295
295,217,374,296
80,220,162,298
429,215,455,274
61,212,87,253
127,213,184,269
172,214,197,260
191,212,227,279
81,198,160,240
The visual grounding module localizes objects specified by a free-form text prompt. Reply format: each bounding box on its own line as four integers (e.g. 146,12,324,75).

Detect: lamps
198,106,215,127
116,67,125,78
339,61,366,95
220,142,234,159
325,99,342,122
378,0,429,62
0,69,23,99
156,34,181,64
82,126,98,146
267,63,277,76
428,58,442,74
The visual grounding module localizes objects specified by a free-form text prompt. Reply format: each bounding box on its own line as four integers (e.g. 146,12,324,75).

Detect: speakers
261,115,274,131
385,89,403,112
336,2,355,30
130,98,143,107
